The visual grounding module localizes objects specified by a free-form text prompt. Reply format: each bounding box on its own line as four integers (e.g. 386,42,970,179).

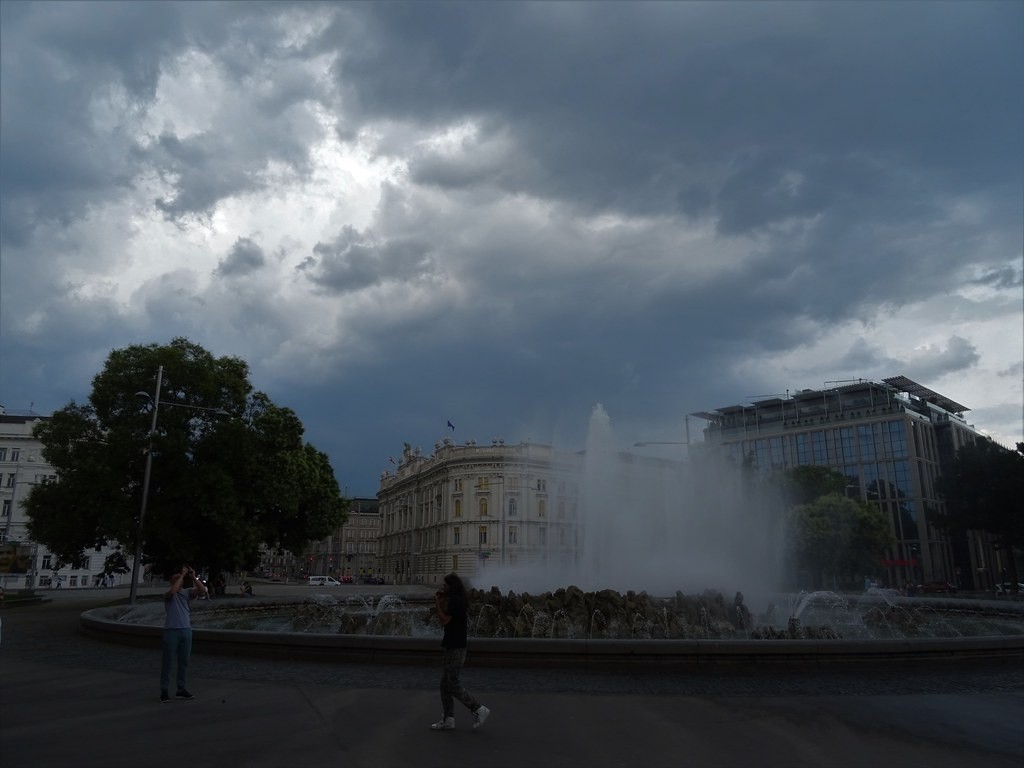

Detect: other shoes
176,689,194,698
473,706,490,729
160,688,172,702
432,713,455,729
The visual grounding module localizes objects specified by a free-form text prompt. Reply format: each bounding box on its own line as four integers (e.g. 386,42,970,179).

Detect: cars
917,581,958,596
307,576,342,586
994,582,1024,595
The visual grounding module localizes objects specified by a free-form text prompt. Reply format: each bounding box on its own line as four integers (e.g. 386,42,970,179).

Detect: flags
448,420,455,431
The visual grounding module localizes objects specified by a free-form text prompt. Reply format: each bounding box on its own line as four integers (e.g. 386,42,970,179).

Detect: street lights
128,400,231,606
128,390,159,604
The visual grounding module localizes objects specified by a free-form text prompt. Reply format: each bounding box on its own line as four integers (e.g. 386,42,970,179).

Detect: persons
94,575,115,589
194,566,212,601
240,581,252,598
159,566,205,703
424,571,490,730
